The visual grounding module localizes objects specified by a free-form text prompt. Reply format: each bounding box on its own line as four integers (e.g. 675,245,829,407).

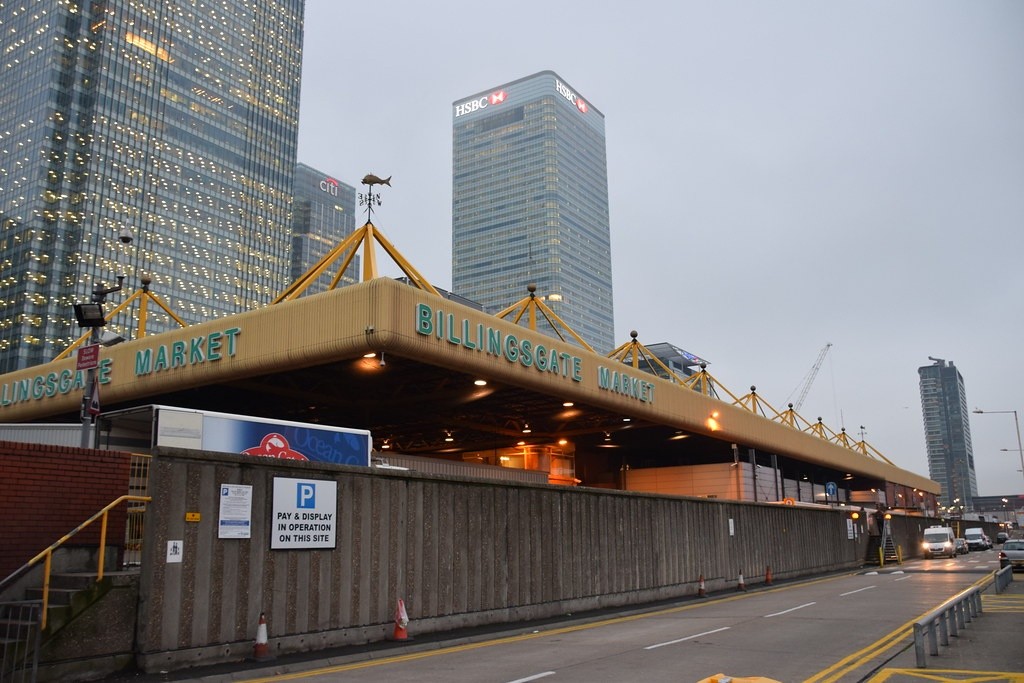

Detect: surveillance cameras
380,360,385,367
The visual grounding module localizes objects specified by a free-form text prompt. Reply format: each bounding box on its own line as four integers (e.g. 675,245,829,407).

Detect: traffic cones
695,575,709,598
248,612,278,662
385,599,414,643
763,565,774,586
735,569,747,592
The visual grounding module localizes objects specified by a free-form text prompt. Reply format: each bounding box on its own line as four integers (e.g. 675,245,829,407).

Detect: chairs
1010,545,1016,549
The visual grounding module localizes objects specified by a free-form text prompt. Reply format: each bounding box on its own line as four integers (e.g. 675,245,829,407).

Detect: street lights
73,301,107,449
972,410,1024,473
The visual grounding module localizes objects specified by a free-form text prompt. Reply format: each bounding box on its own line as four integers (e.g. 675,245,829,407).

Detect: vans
998,533,1009,543
965,528,988,551
923,527,957,559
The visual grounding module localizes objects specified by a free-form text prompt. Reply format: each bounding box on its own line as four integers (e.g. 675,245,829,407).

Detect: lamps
710,409,721,419
380,352,386,366
444,433,454,442
522,424,532,434
604,433,612,441
620,464,630,472
73,303,105,329
381,439,390,449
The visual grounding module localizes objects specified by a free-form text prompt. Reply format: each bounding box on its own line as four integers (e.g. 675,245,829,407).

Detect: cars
986,536,992,547
955,538,968,554
999,538,1024,572
